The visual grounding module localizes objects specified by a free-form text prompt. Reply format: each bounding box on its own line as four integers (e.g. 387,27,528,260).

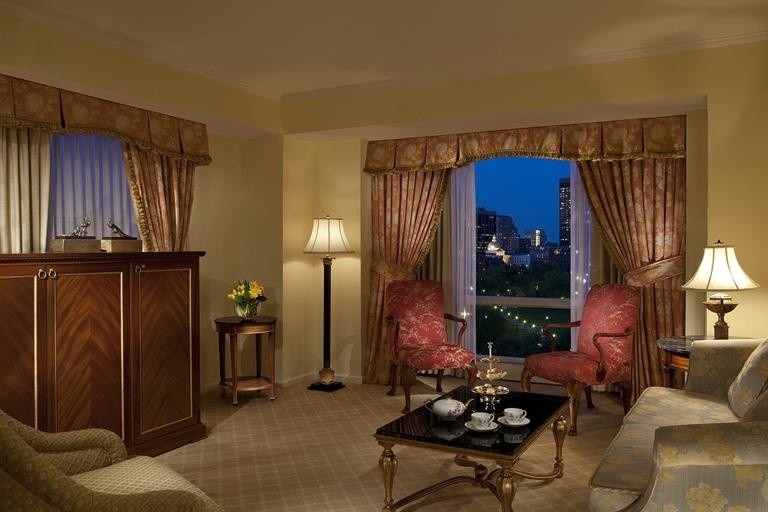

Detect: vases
235,303,259,320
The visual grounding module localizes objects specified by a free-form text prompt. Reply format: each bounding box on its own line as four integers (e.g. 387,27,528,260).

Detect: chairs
385,279,477,414
521,283,641,436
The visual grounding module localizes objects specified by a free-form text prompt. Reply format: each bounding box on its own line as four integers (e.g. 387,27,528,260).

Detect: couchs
587,338,768,512
0,410,224,512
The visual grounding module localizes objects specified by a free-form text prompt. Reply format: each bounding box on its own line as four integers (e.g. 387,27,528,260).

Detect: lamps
302,214,355,392
681,239,760,339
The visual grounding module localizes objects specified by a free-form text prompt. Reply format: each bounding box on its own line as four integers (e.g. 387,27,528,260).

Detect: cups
504,407,527,424
471,438,494,447
471,412,495,429
504,433,527,444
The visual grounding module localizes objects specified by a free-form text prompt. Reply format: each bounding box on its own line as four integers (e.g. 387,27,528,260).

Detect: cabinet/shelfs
132,256,200,444
0,260,132,451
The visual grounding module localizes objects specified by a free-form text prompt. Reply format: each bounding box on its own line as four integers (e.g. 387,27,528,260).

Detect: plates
464,420,498,431
497,416,530,426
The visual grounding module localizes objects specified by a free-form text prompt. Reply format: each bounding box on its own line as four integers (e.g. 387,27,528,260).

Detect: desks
214,315,278,405
656,335,756,387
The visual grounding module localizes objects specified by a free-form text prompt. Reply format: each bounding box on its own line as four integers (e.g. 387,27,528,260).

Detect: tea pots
432,420,467,441
424,396,474,420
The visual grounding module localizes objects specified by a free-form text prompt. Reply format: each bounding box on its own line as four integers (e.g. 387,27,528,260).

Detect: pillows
727,338,768,418
741,389,768,421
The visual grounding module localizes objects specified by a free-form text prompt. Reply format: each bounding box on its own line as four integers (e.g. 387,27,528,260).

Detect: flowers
227,280,267,316
